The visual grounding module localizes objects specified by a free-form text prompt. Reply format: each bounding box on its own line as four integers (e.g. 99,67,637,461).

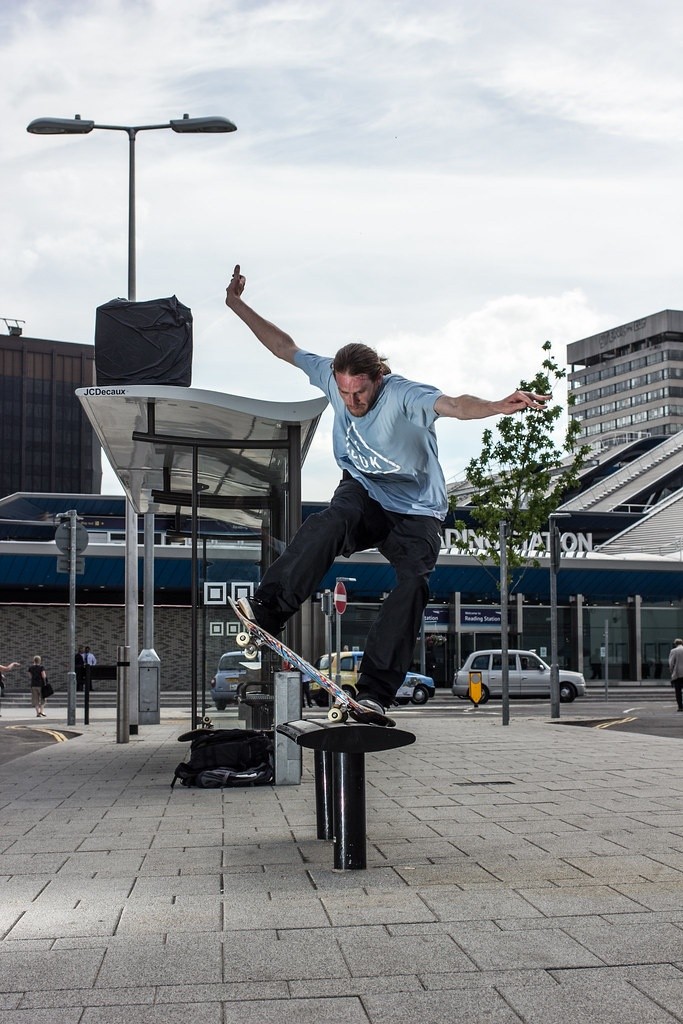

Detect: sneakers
238,595,287,637
355,691,399,715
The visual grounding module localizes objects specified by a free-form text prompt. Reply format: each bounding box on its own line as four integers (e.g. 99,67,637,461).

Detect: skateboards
226,594,399,728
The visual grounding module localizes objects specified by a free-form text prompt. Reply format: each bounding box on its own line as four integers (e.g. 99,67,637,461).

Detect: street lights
548,512,571,716
29,112,239,733
57,511,85,725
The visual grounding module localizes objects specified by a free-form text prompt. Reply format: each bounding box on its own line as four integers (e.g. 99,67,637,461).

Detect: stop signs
334,582,348,613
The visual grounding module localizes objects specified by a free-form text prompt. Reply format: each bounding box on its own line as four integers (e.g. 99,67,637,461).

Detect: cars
309,653,437,705
210,650,260,710
453,650,586,703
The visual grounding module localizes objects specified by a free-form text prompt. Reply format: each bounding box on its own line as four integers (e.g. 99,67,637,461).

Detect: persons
226,264,552,715
669,639,683,711
75,646,97,691
0,662,20,717
27,656,46,717
522,658,537,669
288,654,314,708
427,645,438,687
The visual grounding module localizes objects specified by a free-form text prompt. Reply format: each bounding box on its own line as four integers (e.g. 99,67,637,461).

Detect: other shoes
37,715,41,717
677,709,683,712
39,712,46,716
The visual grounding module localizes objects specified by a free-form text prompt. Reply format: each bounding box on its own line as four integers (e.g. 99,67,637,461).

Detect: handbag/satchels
41,683,53,699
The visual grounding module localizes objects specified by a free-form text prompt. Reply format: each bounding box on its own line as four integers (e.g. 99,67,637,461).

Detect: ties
85,653,88,664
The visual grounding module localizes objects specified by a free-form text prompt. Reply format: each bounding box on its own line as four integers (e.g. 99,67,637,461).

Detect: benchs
276,717,417,870
233,680,275,729
